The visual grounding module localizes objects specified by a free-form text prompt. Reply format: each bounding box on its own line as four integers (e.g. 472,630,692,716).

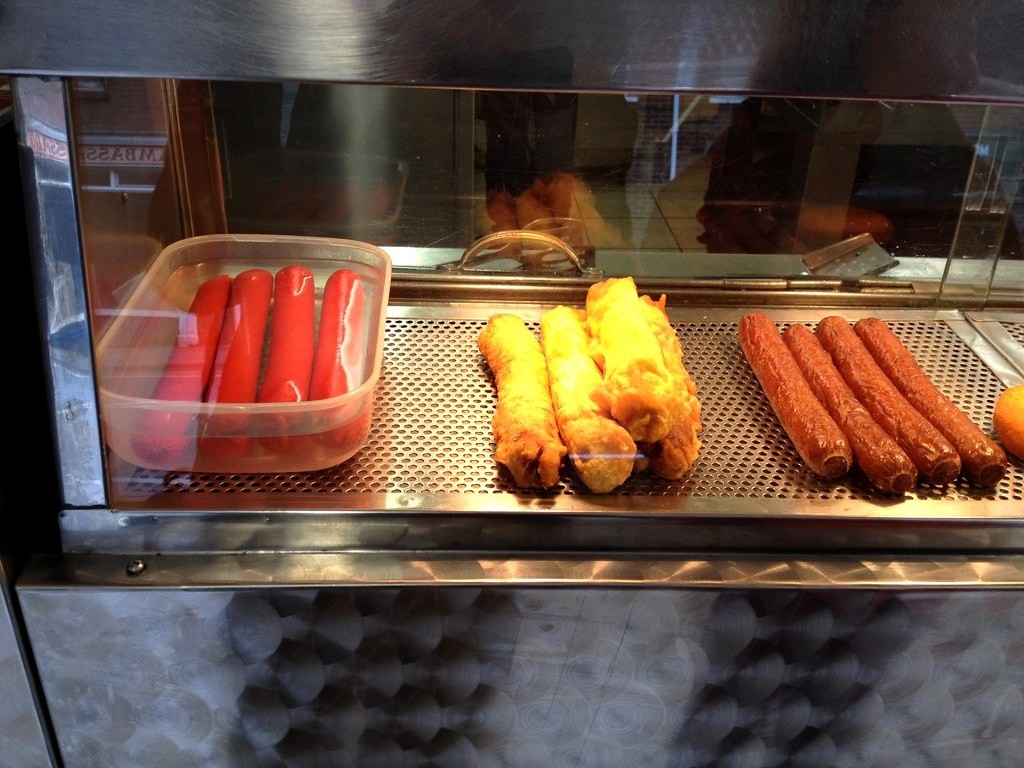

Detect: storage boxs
231,147,412,247
90,233,393,473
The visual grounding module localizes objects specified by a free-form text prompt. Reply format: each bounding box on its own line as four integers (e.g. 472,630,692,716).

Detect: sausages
130,264,369,462
738,312,1009,493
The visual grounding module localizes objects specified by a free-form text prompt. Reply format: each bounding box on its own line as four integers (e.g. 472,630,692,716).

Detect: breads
796,205,896,245
992,384,1024,462
